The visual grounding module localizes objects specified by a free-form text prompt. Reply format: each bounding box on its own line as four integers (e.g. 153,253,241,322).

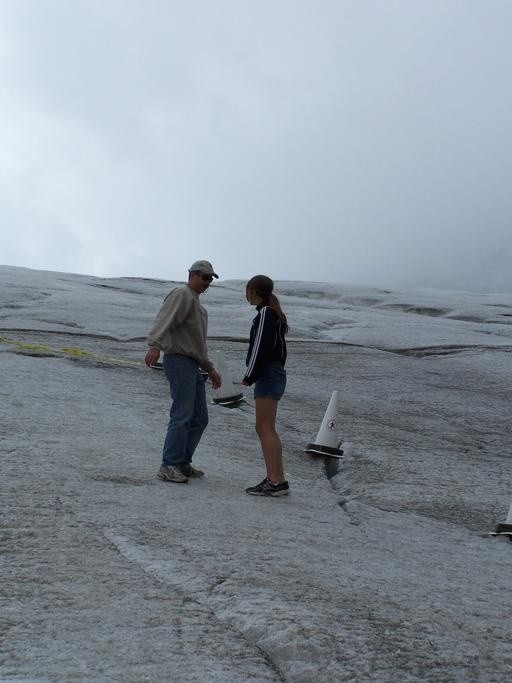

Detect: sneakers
157,463,204,483
245,477,289,497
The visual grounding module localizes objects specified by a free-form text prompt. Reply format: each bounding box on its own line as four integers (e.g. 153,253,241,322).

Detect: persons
144,260,222,483
241,274,290,497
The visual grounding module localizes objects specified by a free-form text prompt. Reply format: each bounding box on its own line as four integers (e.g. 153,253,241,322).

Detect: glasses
195,273,213,282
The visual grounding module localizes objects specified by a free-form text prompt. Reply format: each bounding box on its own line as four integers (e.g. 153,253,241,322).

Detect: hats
187,259,219,279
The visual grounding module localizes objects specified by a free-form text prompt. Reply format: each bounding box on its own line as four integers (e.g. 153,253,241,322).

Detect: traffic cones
486,502,511,536
210,348,247,404
300,388,347,459
